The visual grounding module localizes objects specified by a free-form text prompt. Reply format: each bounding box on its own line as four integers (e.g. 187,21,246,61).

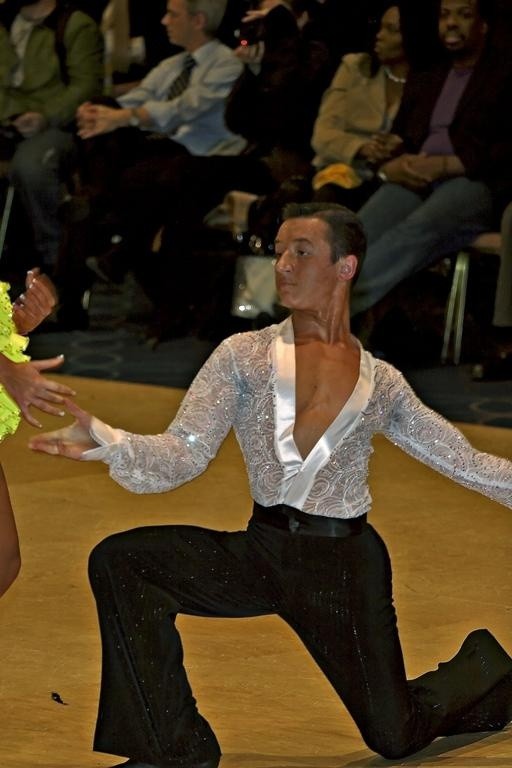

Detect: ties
159,54,195,102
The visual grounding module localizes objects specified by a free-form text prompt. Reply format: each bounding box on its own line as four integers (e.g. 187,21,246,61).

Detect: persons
1,266,79,596
24,199,512,767
0,2,512,337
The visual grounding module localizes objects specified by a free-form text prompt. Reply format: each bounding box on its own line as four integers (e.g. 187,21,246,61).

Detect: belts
253,500,369,540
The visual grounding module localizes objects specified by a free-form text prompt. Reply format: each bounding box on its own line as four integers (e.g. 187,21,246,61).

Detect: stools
440,232,503,367
